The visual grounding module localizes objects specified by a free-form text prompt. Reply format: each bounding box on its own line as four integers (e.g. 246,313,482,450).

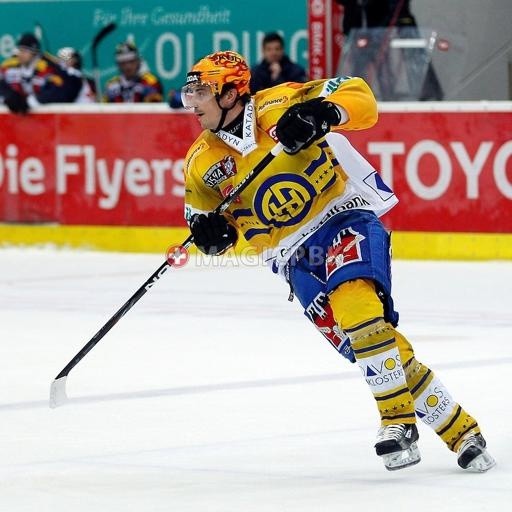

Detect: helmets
18,34,40,52
180,51,251,110
115,43,138,64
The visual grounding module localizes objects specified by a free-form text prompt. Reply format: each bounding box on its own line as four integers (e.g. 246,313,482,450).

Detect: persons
248,29,310,94
0,29,81,111
104,42,163,104
57,47,97,102
182,51,487,469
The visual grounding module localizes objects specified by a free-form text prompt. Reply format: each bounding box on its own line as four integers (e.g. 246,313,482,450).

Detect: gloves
276,96,341,155
189,212,237,255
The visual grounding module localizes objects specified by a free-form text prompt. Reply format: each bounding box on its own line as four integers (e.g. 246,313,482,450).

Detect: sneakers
456,433,486,468
375,422,420,456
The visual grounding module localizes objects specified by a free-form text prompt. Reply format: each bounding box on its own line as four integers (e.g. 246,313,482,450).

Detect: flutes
49,141,285,407
